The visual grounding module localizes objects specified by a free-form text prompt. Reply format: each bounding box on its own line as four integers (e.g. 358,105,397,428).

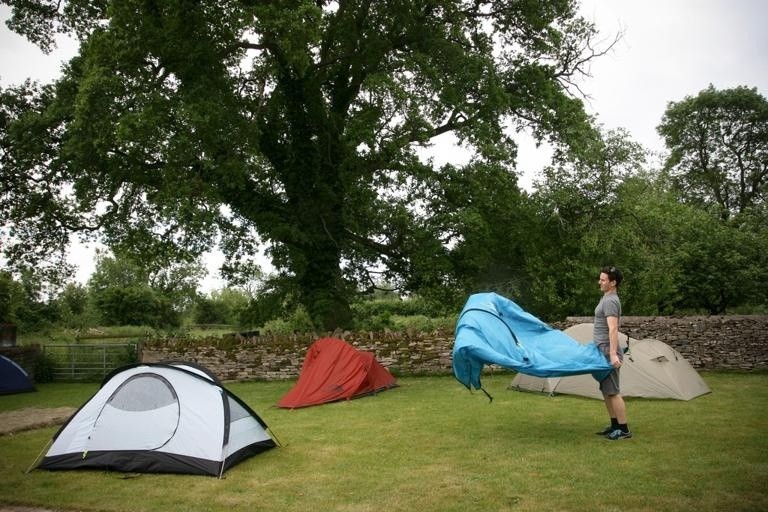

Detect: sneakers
595,426,611,436
607,430,631,441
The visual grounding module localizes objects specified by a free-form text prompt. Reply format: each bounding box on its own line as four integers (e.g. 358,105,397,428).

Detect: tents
506,323,714,402
277,337,398,411
0,352,38,395
24,360,283,482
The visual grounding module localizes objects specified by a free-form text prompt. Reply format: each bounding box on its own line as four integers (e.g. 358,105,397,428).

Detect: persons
594,265,632,440
